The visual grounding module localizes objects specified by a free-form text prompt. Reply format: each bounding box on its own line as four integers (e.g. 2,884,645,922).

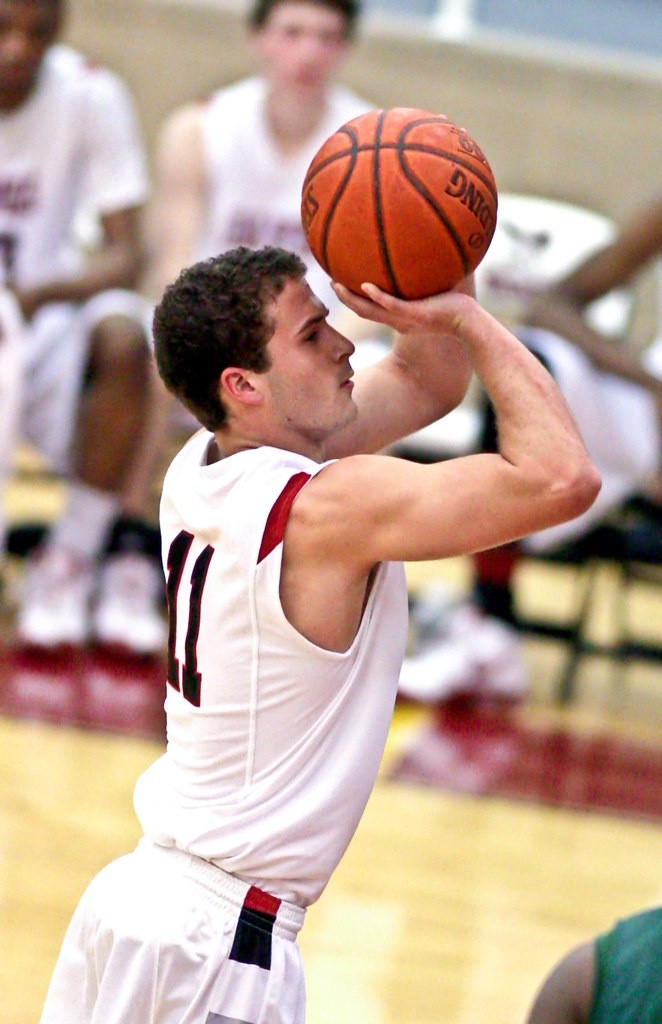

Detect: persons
38,246,605,1024
1,0,662,708
526,907,662,1024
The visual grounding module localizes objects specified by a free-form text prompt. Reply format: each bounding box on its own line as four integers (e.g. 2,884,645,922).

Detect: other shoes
97,554,171,655
17,541,92,646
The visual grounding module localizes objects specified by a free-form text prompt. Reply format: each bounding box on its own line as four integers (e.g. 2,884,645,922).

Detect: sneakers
396,612,527,703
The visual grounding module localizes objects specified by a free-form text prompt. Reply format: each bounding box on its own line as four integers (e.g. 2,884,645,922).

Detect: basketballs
299,107,499,300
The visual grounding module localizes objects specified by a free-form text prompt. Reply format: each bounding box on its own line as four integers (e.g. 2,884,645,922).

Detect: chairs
469,194,629,705
